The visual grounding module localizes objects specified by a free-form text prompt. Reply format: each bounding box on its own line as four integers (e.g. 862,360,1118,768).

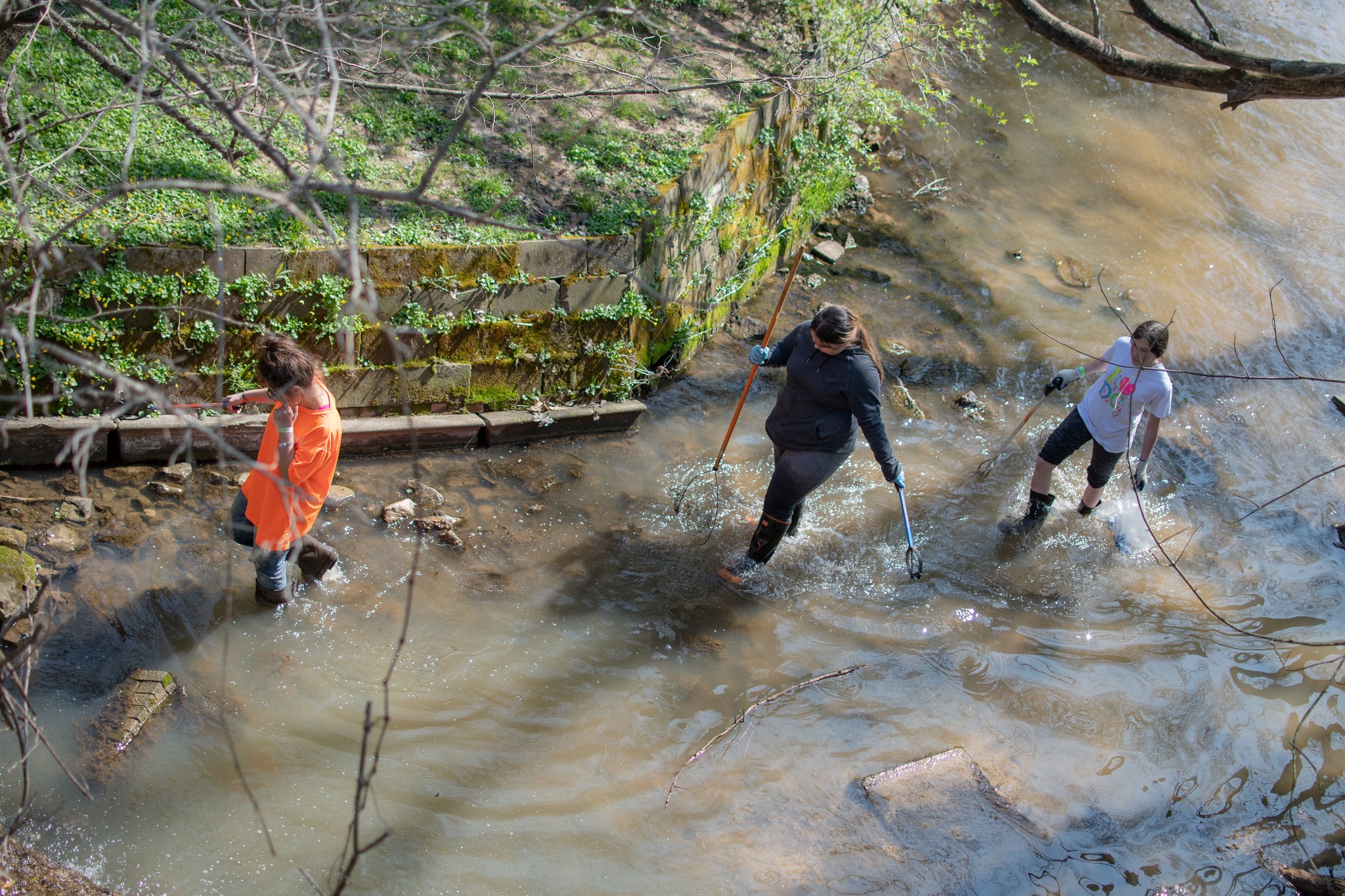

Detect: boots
285,532,339,585
1078,497,1103,515
1000,488,1055,535
256,577,297,605
717,512,792,584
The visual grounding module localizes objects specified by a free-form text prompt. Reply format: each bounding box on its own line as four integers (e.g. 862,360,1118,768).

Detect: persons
221,335,342,603
749,304,905,563
1029,320,1173,515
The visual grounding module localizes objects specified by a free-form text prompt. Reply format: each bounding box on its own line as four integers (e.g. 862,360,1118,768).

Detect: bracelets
1080,366,1085,376
278,441,294,448
278,427,293,432
243,391,250,402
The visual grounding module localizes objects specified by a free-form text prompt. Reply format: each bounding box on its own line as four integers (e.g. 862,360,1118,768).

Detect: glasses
267,388,292,401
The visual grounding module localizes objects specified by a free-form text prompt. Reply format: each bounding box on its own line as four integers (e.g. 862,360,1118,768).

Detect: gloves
1052,366,1083,390
889,466,906,490
749,344,770,365
1130,457,1149,492
